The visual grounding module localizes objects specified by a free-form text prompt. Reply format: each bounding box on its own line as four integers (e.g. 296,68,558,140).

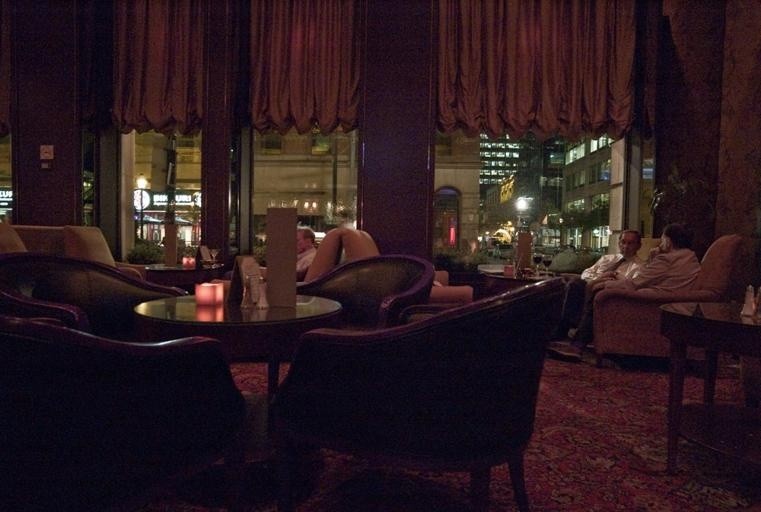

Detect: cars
532,246,545,258
493,244,515,258
553,245,575,255
541,249,557,258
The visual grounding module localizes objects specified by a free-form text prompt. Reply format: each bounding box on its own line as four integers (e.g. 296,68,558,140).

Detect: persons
547,223,703,362
297,228,318,272
555,230,645,335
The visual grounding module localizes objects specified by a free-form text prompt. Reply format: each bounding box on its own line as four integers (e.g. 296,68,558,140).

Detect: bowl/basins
199,259,218,269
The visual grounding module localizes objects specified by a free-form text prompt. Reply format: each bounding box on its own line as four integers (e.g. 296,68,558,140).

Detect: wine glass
207,246,223,269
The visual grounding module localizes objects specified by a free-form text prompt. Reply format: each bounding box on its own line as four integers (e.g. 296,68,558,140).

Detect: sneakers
568,327,593,348
549,345,583,361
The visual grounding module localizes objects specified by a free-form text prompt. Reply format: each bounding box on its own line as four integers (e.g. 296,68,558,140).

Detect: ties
604,258,626,272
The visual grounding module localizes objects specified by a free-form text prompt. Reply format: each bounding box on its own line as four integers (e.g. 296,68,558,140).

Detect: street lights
136,176,145,239
593,228,598,250
558,218,563,247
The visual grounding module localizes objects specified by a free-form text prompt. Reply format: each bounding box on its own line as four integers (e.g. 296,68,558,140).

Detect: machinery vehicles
490,229,511,244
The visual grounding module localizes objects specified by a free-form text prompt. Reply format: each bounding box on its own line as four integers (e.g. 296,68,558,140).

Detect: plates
477,263,557,280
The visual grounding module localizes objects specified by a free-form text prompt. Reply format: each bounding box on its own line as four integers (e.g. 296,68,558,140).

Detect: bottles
533,253,543,278
740,284,756,317
504,258,515,279
756,287,761,308
256,277,269,309
544,255,553,278
240,274,255,312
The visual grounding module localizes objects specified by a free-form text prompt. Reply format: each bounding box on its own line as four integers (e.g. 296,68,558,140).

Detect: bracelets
615,272,619,275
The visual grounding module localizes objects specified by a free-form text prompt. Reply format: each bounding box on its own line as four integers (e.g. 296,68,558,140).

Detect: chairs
1,222,761,511
